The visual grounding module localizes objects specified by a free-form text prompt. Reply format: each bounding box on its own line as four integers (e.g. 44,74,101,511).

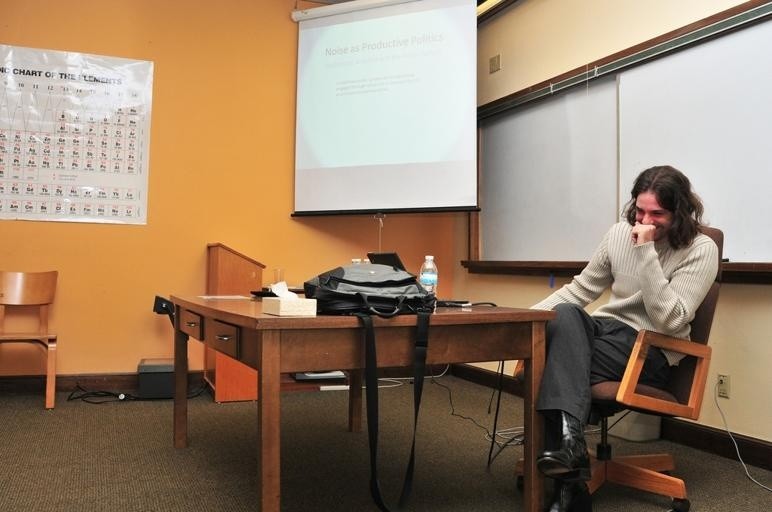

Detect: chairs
0,269,60,411
514,215,724,510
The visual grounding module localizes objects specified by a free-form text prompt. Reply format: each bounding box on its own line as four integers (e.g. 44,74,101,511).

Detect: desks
172,292,559,511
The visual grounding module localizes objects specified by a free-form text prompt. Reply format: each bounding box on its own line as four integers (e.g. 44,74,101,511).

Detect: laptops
367,252,469,307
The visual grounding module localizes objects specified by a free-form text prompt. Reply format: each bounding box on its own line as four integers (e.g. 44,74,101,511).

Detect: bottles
419,254,439,313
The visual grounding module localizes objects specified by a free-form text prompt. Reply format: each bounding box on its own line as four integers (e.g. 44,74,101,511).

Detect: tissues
262,281,317,316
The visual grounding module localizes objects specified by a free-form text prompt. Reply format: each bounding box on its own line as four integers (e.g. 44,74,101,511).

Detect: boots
537,411,591,512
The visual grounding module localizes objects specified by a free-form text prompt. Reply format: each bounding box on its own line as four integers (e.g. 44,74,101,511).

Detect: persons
529,163,719,512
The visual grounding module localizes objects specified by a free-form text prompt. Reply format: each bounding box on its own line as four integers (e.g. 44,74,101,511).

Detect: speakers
138,363,175,400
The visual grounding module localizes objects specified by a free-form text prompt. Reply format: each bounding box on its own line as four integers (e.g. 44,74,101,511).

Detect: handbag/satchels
304,263,438,317
